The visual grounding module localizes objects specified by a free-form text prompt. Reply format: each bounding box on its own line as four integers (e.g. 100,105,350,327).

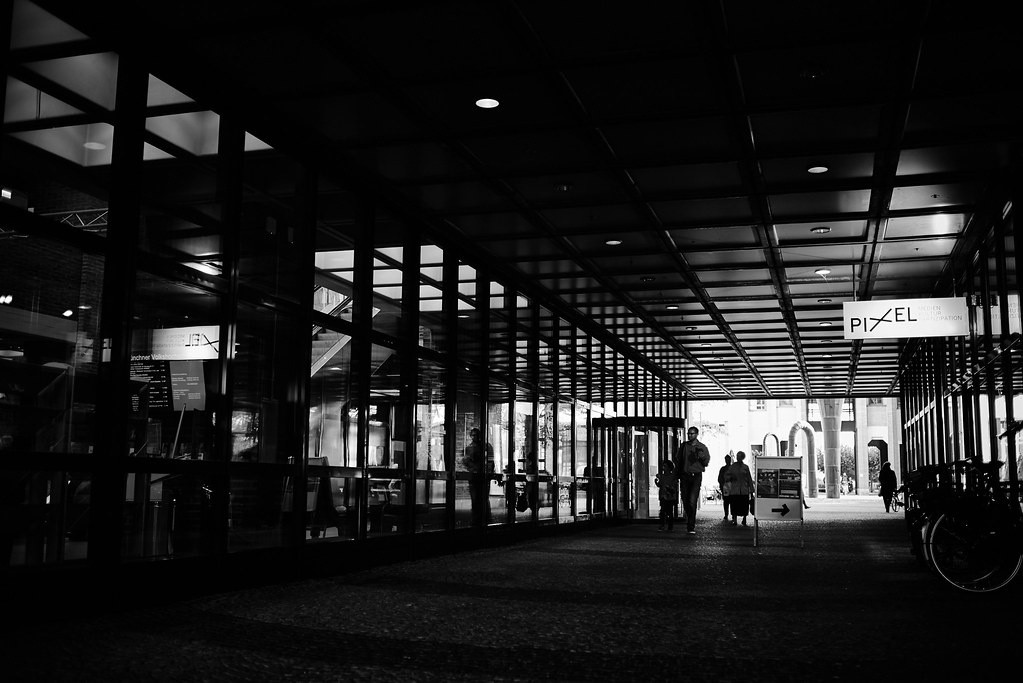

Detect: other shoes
742,521,746,525
667,528,674,533
732,519,737,526
685,529,696,535
724,517,727,519
657,527,665,532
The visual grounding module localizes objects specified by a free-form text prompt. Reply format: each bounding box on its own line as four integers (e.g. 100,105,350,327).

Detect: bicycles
890,418,1023,594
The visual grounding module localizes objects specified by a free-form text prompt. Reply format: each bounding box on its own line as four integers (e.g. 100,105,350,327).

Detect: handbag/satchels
748,492,755,515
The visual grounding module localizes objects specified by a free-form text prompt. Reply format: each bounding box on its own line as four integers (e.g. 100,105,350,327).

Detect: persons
655,427,710,535
719,451,754,528
464,428,495,524
580,456,602,513
879,461,897,513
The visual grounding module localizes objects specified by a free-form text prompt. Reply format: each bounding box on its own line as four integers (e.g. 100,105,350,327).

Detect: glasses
687,433,697,434
471,434,477,436
